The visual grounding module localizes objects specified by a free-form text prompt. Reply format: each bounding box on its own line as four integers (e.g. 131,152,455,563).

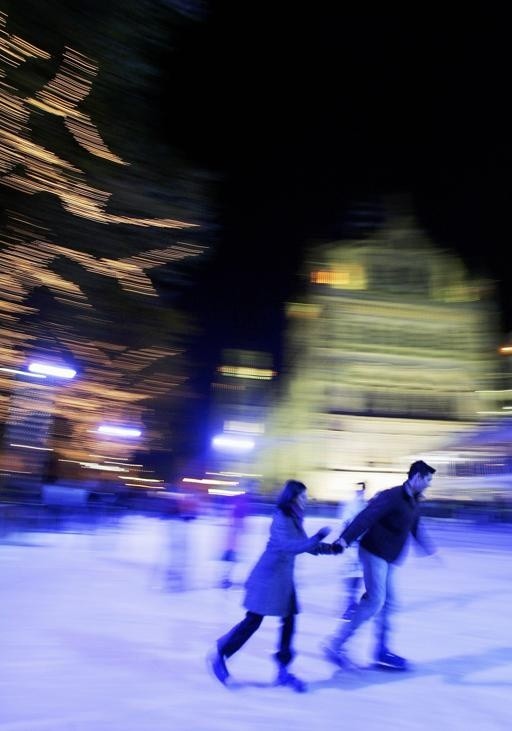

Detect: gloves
316,526,331,539
332,544,343,555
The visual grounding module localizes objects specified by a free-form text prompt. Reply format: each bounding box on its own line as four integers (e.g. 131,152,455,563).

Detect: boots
327,622,354,652
273,666,304,692
342,603,357,619
206,646,230,682
375,644,405,666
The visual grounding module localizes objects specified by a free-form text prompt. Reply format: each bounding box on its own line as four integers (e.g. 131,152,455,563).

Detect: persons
209,460,439,693
